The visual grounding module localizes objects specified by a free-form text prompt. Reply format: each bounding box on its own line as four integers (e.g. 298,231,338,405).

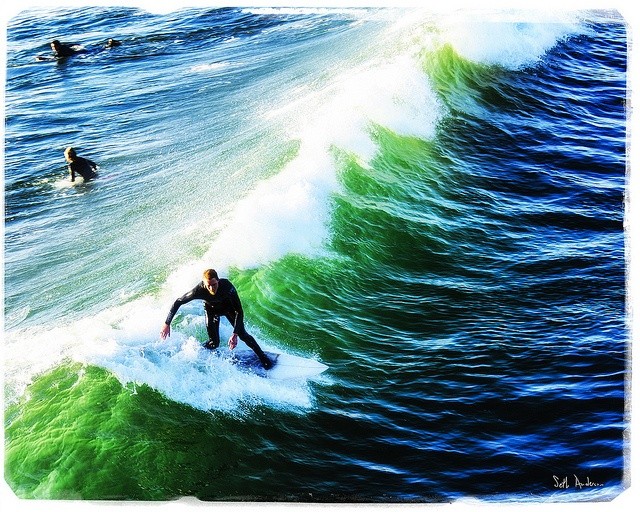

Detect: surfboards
232,351,329,380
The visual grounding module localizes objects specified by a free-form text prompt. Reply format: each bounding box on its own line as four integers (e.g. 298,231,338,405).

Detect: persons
63,147,96,182
51,38,122,59
160,269,272,368
